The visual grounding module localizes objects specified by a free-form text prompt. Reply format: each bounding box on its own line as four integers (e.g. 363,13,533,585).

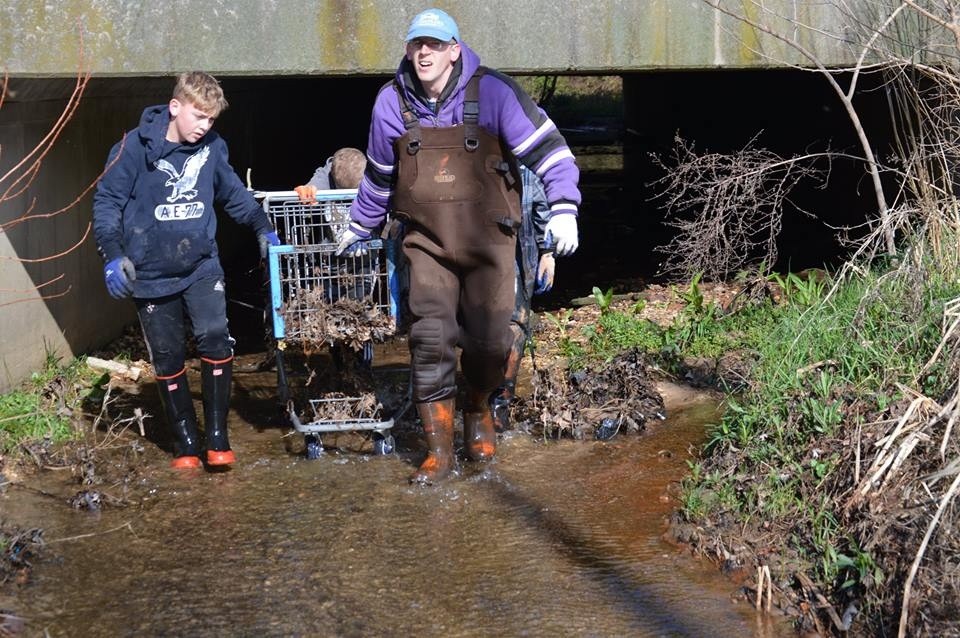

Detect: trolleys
251,189,414,460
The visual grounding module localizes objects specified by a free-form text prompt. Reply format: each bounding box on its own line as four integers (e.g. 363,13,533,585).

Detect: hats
404,9,460,43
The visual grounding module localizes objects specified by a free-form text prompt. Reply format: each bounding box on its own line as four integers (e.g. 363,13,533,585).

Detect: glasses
409,37,451,51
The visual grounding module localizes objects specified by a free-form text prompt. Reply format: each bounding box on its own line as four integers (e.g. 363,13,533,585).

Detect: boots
155,367,199,469
493,349,519,432
201,347,236,464
462,390,497,460
408,398,454,485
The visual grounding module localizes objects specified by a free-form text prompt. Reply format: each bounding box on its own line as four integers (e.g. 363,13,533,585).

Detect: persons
493,160,555,430
93,73,280,476
292,148,372,396
335,7,582,484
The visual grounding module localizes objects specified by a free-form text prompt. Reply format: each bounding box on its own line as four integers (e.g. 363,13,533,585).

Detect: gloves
104,256,135,300
534,254,555,292
295,184,318,205
260,233,281,258
544,204,579,256
335,222,372,257
